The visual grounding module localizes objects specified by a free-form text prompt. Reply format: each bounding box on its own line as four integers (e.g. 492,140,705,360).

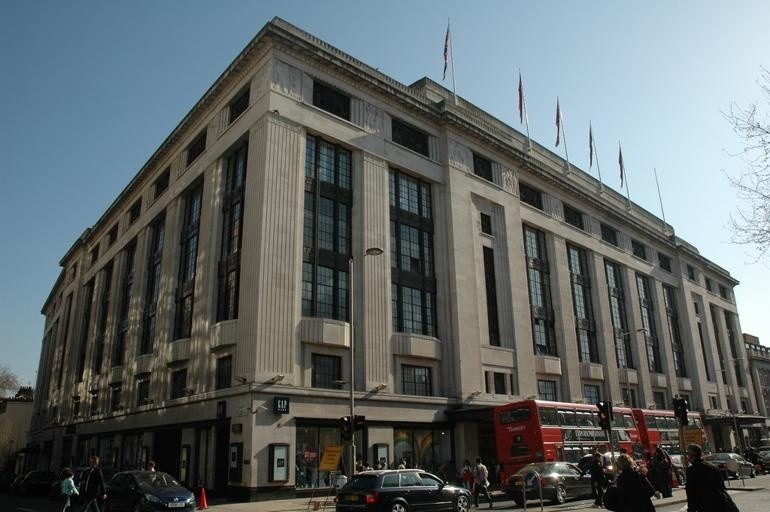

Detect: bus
495,404,704,490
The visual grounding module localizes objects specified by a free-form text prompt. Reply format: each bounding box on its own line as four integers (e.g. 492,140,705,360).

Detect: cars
0,470,196,512
336,469,472,512
494,452,770,506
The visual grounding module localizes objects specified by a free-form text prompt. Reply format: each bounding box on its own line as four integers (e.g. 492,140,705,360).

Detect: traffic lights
355,415,365,431
340,416,352,440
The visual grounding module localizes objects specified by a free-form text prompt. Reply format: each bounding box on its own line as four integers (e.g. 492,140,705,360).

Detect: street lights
348,248,384,477
620,329,648,408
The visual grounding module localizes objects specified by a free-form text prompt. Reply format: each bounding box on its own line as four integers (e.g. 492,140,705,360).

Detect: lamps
267,375,285,385
72,387,193,419
372,384,387,393
468,391,481,401
233,376,247,385
527,394,760,416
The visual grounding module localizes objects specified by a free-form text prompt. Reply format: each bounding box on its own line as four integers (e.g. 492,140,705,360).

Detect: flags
555,95,564,148
443,17,453,80
617,140,627,187
587,120,597,170
517,67,527,125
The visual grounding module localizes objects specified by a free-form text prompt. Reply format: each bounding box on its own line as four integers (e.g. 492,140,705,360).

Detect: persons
588,441,674,508
355,456,408,473
684,442,741,512
55,469,79,511
459,458,473,490
52,459,73,487
472,455,493,508
142,461,158,484
735,446,766,475
615,452,658,512
78,454,108,511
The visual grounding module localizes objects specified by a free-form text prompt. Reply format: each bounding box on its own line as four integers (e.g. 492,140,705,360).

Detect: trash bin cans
335,475,347,495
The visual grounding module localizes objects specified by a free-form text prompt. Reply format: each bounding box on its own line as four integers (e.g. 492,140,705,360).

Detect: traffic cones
199,487,208,510
